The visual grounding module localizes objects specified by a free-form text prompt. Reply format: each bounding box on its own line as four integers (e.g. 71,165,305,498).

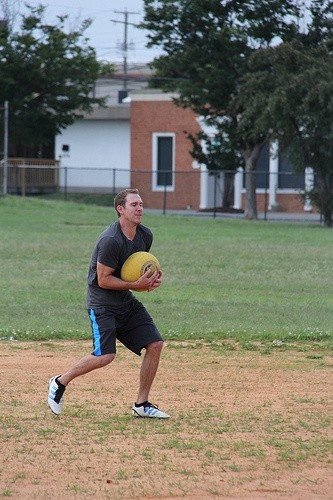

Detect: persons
47,189,171,420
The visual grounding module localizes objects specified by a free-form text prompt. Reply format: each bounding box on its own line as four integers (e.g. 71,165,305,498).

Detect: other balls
122,251,162,293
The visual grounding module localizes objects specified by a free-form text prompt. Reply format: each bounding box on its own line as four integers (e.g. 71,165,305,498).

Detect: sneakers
132,402,171,418
48,374,63,415
